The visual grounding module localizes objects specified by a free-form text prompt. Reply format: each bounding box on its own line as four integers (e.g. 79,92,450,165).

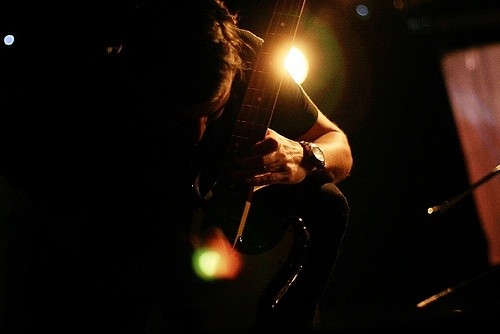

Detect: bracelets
300,141,323,176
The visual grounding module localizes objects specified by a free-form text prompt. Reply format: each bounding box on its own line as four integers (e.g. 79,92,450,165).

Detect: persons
151,1,354,334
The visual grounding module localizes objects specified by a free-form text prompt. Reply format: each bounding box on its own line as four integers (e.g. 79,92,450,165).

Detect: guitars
144,0,313,334
